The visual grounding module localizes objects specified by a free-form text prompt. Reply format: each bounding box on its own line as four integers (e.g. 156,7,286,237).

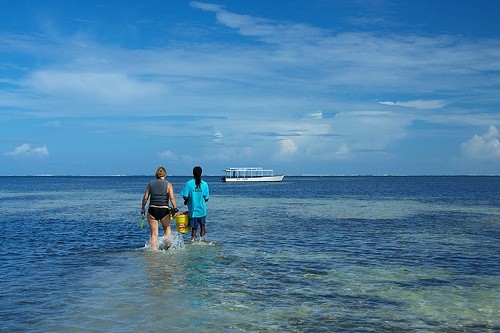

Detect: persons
141,167,177,250
180,166,209,240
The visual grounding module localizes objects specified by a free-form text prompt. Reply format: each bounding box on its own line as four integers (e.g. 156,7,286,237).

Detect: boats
225,167,286,182
